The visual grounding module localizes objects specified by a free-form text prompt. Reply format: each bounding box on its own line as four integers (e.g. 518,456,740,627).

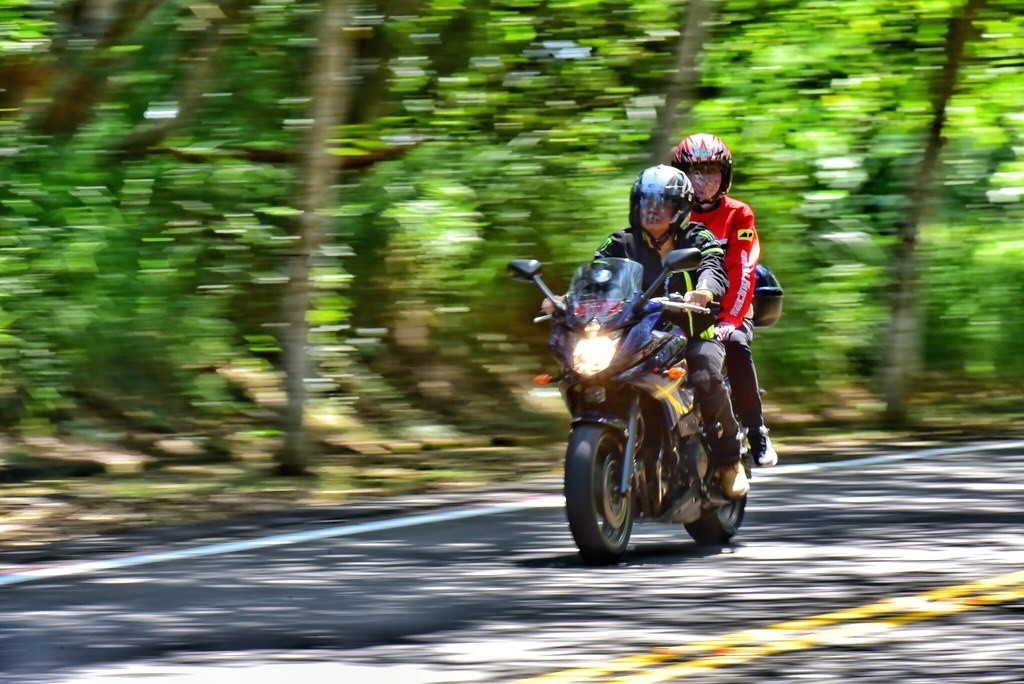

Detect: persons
670,134,779,467
541,163,750,498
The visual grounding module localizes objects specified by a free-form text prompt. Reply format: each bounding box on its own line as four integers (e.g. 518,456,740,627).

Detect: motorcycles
506,248,786,572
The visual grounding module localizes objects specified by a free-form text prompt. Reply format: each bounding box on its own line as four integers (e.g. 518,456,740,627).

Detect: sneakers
746,425,778,467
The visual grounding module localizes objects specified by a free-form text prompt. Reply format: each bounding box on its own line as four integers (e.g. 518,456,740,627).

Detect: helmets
629,165,696,232
670,133,733,199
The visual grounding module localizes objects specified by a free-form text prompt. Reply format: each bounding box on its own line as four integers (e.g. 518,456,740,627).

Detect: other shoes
719,460,749,497
